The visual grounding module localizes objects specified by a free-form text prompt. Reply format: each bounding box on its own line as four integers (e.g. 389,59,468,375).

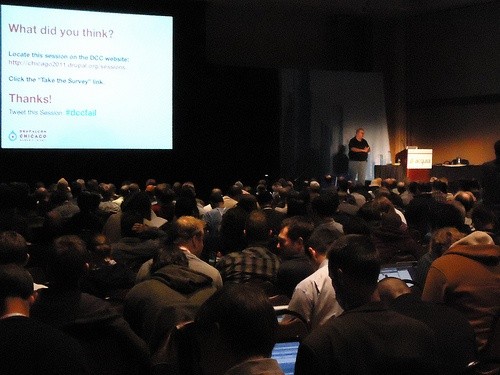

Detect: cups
456,157,461,164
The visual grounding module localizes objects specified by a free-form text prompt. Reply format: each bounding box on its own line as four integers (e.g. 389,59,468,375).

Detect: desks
374,165,500,187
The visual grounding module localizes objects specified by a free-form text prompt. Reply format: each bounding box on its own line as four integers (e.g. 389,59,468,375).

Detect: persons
0,140,500,375
348,128,370,185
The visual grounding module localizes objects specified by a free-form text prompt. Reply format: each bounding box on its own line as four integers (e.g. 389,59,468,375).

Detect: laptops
273,305,289,322
271,337,303,375
377,267,416,288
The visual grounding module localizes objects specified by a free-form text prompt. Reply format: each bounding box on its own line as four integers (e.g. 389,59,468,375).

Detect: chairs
269,309,308,375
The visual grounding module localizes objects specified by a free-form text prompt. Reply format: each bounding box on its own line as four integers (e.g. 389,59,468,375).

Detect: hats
234,182,243,189
